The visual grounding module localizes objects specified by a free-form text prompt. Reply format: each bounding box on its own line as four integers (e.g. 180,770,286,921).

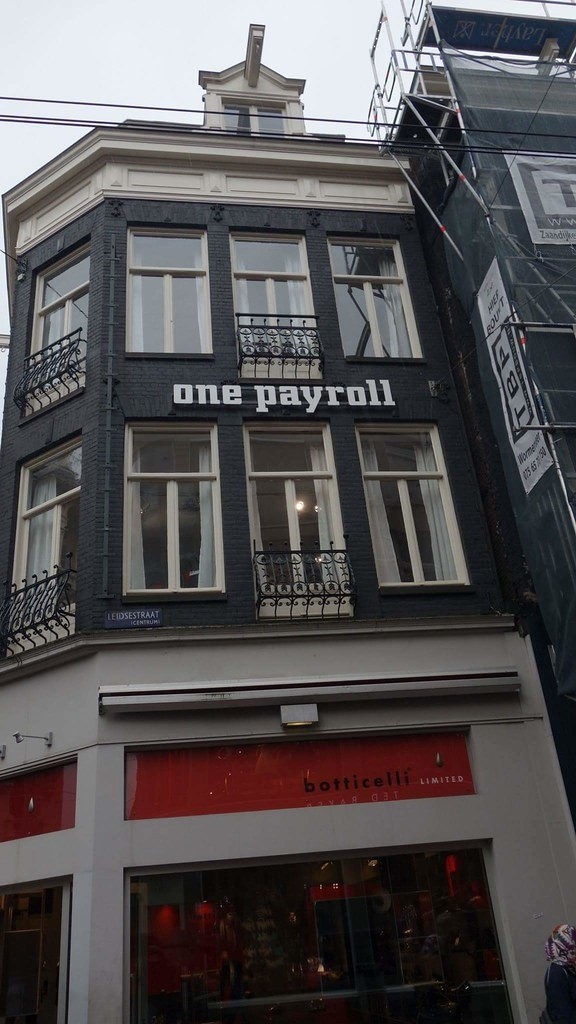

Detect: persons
544,923,576,1024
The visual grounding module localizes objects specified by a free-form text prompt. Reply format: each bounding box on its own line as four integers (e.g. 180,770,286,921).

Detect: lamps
318,861,329,871
367,856,378,867
279,703,318,729
13,731,53,745
